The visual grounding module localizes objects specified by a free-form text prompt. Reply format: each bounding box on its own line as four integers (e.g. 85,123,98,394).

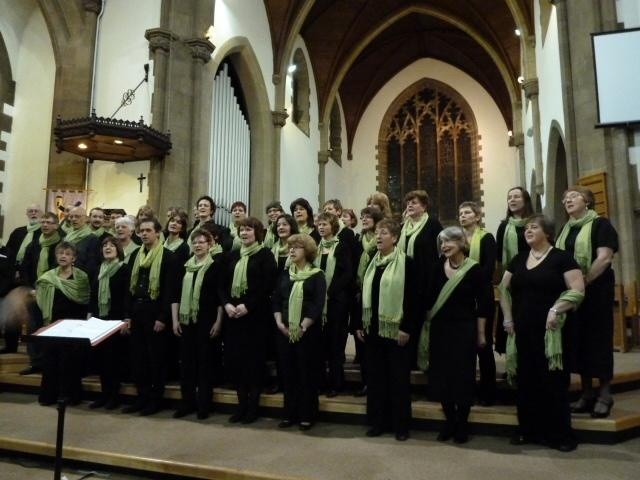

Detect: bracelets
550,308,560,314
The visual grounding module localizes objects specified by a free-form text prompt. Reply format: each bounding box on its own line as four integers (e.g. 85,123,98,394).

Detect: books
38,316,124,344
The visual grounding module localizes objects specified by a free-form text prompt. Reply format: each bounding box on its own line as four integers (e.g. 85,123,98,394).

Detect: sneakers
173,408,193,417
228,414,243,423
196,411,208,419
394,432,409,441
140,407,159,416
573,395,592,412
279,419,296,427
106,402,121,411
299,420,313,430
241,417,257,424
89,399,105,408
366,426,386,437
592,392,613,419
122,403,139,414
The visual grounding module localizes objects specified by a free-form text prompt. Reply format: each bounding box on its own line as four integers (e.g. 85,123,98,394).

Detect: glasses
193,241,207,245
289,245,304,250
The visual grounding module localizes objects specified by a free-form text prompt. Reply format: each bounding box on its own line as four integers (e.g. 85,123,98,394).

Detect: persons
351,218,424,442
272,233,327,431
424,225,491,444
397,189,444,271
115,217,141,264
498,213,586,451
61,206,100,286
1,203,44,354
131,205,155,246
35,241,92,406
19,211,67,375
262,201,286,248
313,212,350,398
322,198,355,249
218,216,277,424
87,207,114,241
290,198,322,246
494,186,535,379
353,191,392,364
186,195,233,257
458,200,496,407
270,213,298,277
108,209,126,237
554,187,620,420
169,229,223,419
120,218,177,417
354,207,384,397
163,212,190,263
86,236,128,410
226,201,247,251
340,208,358,229
